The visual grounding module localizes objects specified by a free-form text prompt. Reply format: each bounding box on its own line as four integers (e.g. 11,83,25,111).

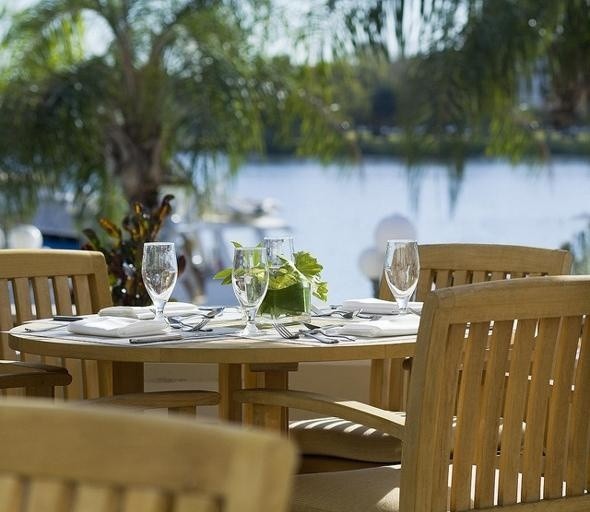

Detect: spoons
312,306,365,320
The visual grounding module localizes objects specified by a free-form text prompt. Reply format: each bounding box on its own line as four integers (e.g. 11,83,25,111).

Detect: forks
270,322,339,347
150,302,225,332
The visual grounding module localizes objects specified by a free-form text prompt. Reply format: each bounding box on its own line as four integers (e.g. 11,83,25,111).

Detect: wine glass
229,246,271,338
382,238,421,316
141,240,178,330
264,231,297,265
